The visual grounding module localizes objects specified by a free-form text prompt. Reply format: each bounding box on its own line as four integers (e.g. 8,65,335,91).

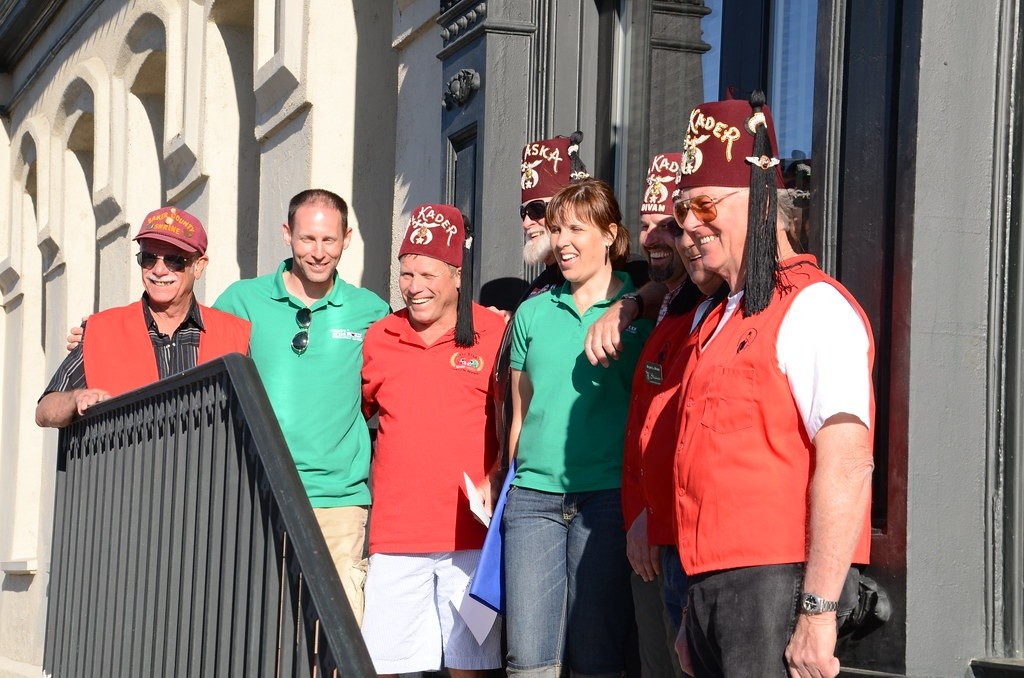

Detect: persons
503,91,877,678
66,189,515,677
34,206,250,430
361,201,508,678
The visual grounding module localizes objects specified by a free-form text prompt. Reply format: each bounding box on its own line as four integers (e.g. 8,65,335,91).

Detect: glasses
672,190,742,228
519,199,550,222
291,306,312,356
135,251,199,272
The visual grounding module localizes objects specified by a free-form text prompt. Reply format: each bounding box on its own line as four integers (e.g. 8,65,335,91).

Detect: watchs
799,593,838,615
621,293,644,319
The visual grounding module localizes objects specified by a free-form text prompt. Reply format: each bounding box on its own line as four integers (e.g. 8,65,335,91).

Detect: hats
398,203,476,348
640,152,682,215
681,89,786,318
132,206,208,255
520,131,590,204
